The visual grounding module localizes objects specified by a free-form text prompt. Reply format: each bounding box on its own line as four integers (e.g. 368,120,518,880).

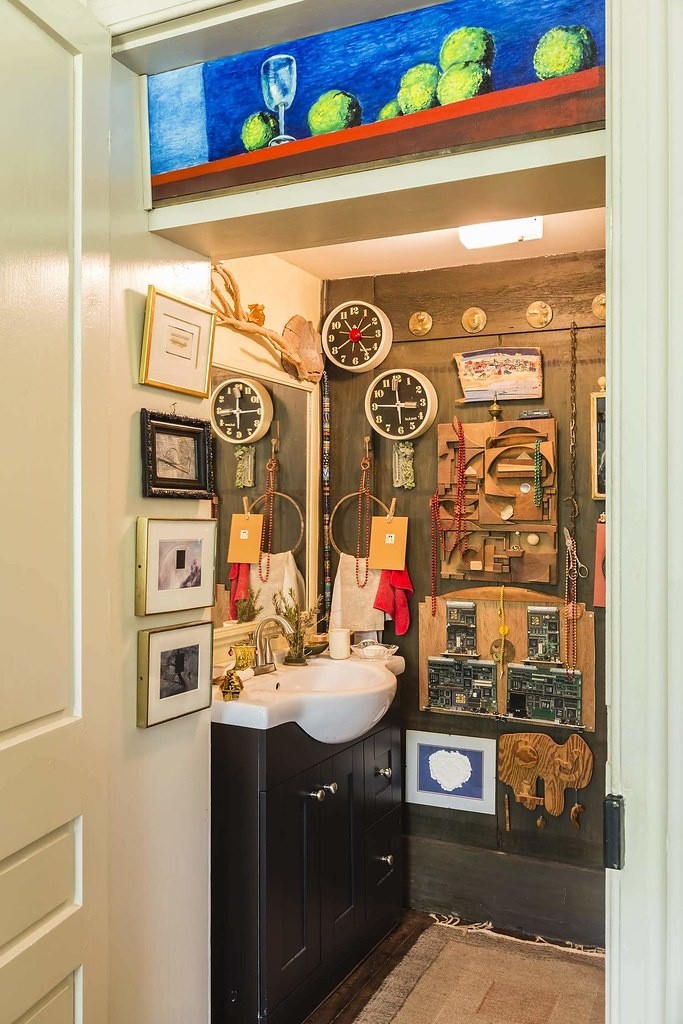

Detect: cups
329,629,350,660
223,620,238,627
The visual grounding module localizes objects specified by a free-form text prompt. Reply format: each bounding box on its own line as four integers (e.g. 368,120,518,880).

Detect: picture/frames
591,390,607,498
134,408,218,501
135,620,217,729
405,730,498,815
133,516,227,618
140,284,219,395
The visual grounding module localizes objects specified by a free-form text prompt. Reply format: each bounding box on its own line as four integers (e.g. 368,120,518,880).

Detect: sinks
254,659,397,744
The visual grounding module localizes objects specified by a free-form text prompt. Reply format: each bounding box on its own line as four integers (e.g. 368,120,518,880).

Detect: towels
228,563,250,620
372,565,413,635
328,552,385,632
249,550,305,620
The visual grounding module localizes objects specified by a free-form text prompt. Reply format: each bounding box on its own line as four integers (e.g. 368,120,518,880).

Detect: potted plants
274,590,328,665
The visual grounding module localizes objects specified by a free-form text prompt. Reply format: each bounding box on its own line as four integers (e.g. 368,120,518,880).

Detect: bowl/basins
305,641,329,657
350,639,399,660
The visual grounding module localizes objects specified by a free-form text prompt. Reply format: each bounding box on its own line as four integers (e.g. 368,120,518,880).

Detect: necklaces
564,537,577,680
259,464,275,582
533,440,543,508
430,420,465,618
355,460,369,587
210,493,219,600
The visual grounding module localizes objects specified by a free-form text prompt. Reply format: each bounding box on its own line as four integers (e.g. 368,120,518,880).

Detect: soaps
363,645,388,657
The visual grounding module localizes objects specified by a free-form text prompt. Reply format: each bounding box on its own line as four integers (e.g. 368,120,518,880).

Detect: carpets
355,910,606,1024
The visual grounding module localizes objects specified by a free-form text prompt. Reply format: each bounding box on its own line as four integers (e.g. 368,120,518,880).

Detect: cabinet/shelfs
210,713,417,1022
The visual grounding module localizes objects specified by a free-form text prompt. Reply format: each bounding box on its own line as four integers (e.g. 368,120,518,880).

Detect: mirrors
216,370,318,631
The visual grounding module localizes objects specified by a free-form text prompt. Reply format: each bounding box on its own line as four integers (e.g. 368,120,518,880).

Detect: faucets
254,615,295,667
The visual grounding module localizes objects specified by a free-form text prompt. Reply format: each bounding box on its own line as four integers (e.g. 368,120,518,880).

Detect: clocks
364,368,441,441
205,378,274,446
321,299,393,375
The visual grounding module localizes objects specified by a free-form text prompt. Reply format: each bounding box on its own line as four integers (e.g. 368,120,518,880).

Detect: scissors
563,526,589,581
